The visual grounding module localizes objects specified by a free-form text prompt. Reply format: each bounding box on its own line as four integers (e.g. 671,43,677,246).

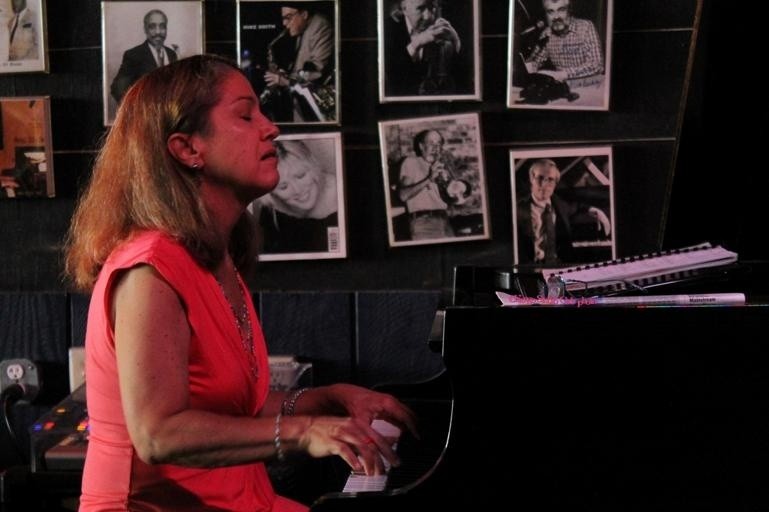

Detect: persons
517,159,611,267
64,55,419,512
526,0,604,82
7,1,39,61
110,10,178,109
261,1,334,122
395,128,471,241
386,0,461,95
258,140,338,255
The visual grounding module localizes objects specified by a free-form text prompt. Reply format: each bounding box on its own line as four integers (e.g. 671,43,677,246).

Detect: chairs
0,382,47,468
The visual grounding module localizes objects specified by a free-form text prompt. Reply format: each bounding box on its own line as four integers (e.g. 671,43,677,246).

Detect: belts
412,210,447,217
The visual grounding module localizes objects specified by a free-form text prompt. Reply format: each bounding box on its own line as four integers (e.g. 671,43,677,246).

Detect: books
542,241,738,288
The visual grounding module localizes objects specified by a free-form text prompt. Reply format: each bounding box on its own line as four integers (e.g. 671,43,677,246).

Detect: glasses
547,275,648,302
282,12,299,20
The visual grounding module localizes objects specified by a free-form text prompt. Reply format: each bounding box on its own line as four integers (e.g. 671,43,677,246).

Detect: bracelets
280,390,293,417
287,388,309,417
273,413,285,462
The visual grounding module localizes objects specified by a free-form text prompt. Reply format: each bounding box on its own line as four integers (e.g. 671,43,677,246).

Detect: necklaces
214,262,254,353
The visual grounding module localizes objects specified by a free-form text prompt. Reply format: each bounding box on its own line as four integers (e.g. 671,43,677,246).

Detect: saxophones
258,27,294,103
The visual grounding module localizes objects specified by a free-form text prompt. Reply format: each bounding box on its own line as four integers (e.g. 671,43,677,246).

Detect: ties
10,17,20,45
156,48,164,63
541,204,556,255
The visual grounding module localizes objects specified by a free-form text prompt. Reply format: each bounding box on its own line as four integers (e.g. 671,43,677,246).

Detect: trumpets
434,150,471,206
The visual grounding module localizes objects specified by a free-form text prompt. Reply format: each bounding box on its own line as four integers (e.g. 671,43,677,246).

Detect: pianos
316,261,768,509
